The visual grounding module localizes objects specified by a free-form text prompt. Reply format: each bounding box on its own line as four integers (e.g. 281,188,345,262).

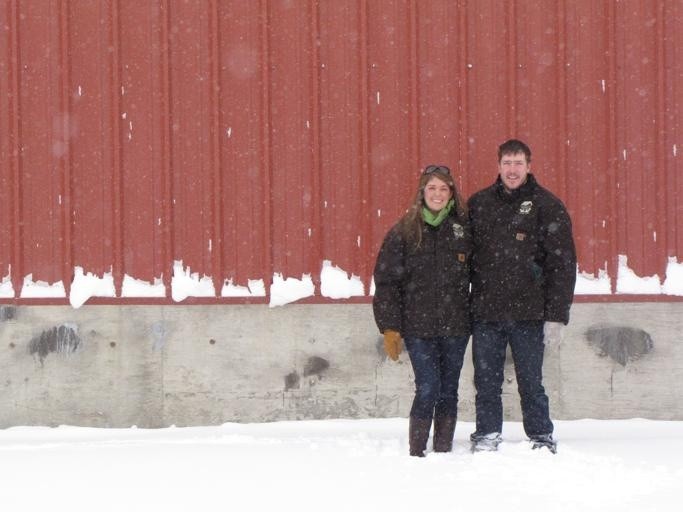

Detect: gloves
542,321,563,346
383,330,402,361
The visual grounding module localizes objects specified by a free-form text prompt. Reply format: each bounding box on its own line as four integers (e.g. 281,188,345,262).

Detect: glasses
423,166,450,175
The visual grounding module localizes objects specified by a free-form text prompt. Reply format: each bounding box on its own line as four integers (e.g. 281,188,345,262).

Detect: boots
408,414,457,457
470,429,502,453
526,432,556,453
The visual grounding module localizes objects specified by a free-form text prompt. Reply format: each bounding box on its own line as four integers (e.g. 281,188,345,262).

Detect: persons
459,139,576,454
372,163,473,458
